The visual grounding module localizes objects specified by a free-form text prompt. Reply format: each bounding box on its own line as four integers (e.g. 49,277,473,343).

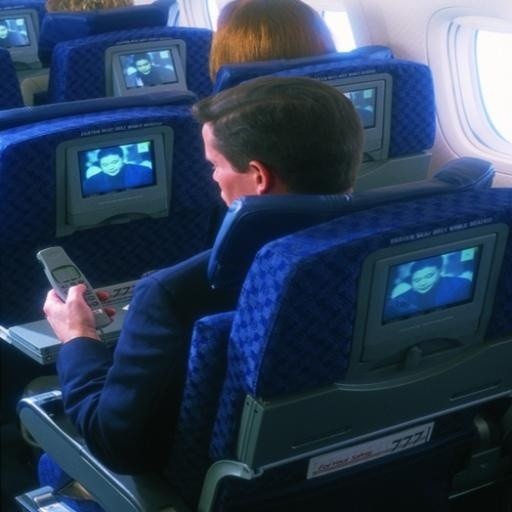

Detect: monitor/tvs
113,45,187,98
332,79,386,151
363,231,498,359
66,133,168,228
0,12,37,56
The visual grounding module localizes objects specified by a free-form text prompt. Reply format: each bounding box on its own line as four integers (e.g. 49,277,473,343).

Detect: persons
0,20,28,47
384,257,472,321
126,54,177,87
20,0,134,104
20,76,363,477
82,147,153,196
208,0,338,81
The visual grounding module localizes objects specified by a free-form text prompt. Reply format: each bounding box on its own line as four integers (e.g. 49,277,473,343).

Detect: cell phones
36,245,112,331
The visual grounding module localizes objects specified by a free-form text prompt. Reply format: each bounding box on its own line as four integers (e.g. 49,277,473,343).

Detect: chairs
0,3,509,512
